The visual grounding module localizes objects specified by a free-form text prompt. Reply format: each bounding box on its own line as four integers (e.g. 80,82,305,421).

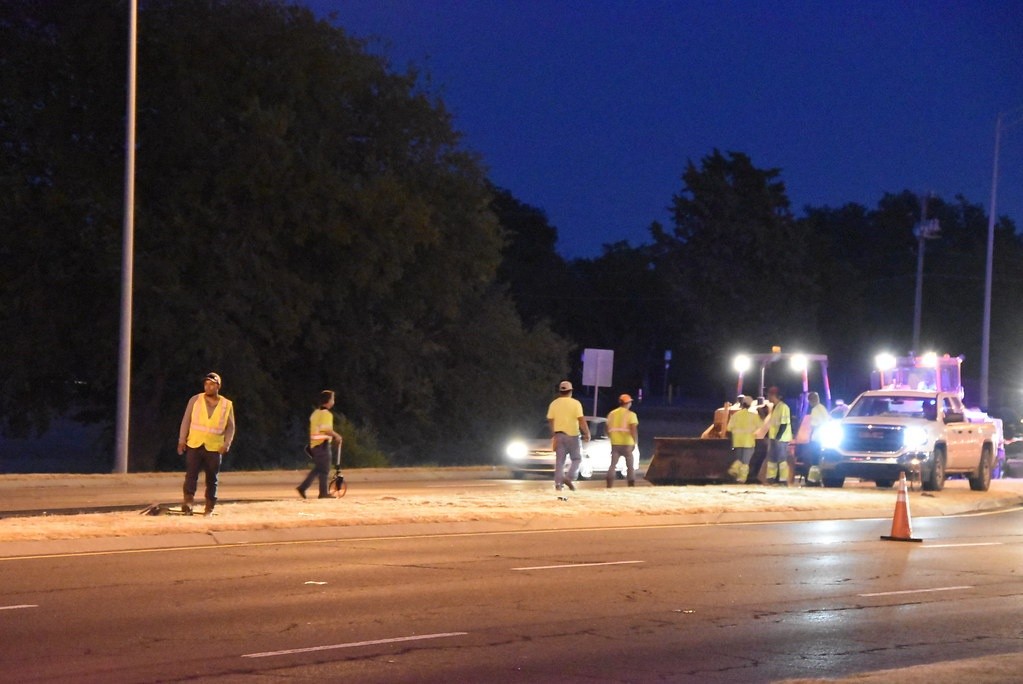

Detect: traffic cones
879,470,926,542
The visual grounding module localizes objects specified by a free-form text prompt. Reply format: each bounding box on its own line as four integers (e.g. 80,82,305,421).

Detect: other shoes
563,478,575,490
296,486,306,499
806,481,822,487
773,481,790,487
317,492,337,498
607,478,613,487
628,480,634,486
764,478,778,486
556,485,563,490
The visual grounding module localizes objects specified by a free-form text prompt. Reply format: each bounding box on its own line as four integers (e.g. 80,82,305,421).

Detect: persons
727,393,774,484
177,373,235,516
765,387,793,488
296,391,342,501
546,382,591,500
803,391,829,487
606,394,640,488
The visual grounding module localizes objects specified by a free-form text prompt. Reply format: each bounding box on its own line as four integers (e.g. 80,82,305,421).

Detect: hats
619,394,634,403
203,373,221,384
559,381,573,391
768,386,778,393
743,396,753,405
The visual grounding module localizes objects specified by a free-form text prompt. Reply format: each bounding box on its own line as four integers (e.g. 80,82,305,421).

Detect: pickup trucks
818,391,998,494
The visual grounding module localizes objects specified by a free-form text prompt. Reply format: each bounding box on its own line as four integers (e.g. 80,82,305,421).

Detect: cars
504,417,640,481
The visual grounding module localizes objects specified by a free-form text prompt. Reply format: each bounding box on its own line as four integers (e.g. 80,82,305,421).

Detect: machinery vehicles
644,352,834,486
874,351,1004,471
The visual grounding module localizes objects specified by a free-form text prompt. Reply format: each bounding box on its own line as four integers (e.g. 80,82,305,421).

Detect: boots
204,497,218,519
183,494,194,515
